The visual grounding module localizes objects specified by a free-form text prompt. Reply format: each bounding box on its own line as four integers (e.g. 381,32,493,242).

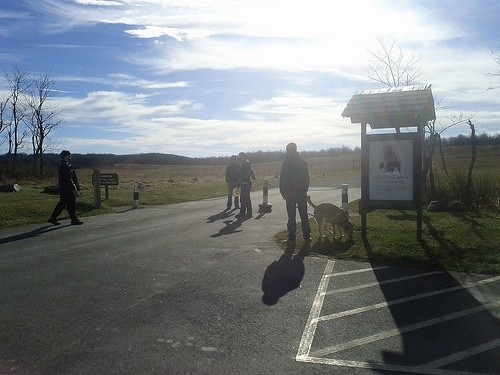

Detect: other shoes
48,217,60,225
303,235,313,242
288,236,296,242
244,215,252,219
235,213,246,218
71,220,82,226
227,206,231,209
235,207,240,209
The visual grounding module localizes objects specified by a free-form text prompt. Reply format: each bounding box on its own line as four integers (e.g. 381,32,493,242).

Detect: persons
226,155,241,209
279,142,312,244
235,152,255,218
47,150,84,225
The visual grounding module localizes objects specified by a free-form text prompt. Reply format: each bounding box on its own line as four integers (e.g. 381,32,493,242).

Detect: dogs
306,194,355,243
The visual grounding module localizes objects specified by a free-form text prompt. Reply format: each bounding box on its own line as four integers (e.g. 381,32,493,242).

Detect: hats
286,142,297,151
233,188,241,196
